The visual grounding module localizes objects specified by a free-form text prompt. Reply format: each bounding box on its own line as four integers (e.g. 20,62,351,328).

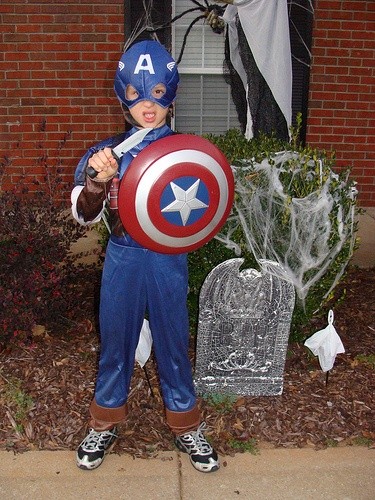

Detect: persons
70,40,221,474
202,0,292,143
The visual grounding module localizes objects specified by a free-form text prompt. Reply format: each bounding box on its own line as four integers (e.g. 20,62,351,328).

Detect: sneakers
76,425,120,470
175,421,220,473
